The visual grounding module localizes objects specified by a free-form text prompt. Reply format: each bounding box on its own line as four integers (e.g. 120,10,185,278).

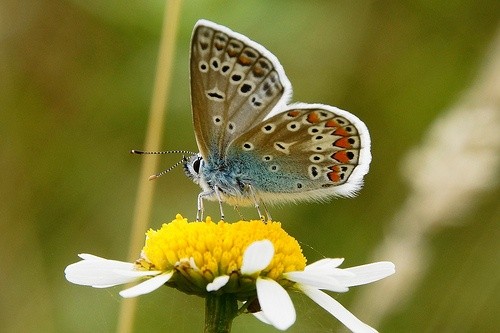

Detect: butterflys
131,18,373,223
